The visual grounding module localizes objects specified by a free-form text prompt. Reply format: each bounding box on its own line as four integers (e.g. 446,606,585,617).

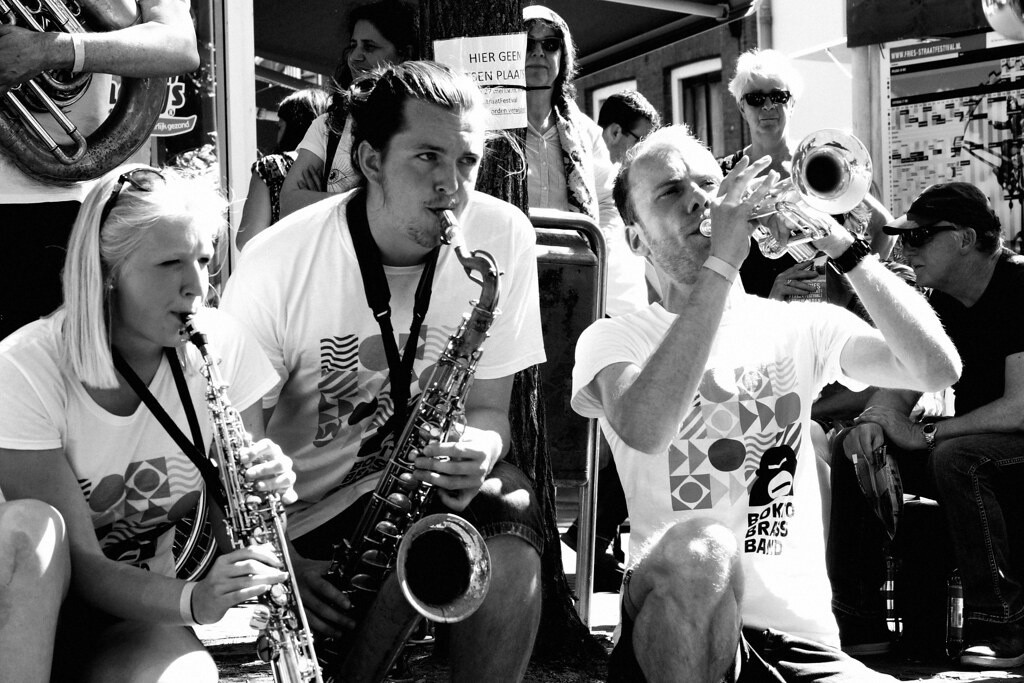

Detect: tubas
0,0,168,182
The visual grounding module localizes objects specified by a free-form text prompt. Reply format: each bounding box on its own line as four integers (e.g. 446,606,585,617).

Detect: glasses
901,225,963,248
99,168,166,241
740,90,791,106
526,37,564,52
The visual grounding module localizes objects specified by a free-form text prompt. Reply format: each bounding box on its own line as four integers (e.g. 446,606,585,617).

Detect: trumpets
698,128,873,263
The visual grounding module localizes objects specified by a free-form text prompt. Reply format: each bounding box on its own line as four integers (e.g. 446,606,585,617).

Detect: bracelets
827,229,871,275
180,582,202,627
71,32,85,74
701,254,741,284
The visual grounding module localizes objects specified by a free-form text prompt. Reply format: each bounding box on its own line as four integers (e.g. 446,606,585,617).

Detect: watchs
922,423,938,451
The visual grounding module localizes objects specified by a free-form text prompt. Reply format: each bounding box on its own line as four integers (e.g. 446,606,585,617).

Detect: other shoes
839,609,892,656
960,621,1024,667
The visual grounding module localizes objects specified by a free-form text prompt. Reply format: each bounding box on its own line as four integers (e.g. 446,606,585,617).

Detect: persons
0,0,1024,683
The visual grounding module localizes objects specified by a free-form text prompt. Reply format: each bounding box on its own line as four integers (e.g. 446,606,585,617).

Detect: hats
882,182,996,236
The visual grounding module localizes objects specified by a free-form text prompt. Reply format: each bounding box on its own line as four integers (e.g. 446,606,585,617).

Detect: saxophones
178,312,324,683
317,210,504,683
